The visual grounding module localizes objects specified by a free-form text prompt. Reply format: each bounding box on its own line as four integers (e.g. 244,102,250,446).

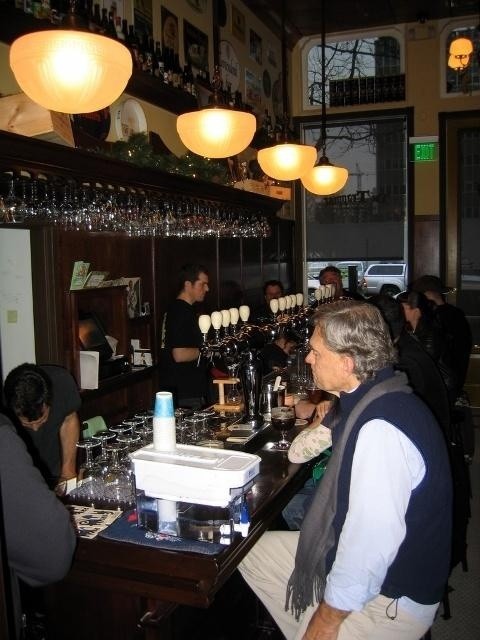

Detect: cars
308,262,407,301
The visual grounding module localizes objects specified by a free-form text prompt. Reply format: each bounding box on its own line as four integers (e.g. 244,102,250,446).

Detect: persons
158,264,217,410
286,292,455,469
395,289,465,407
229,298,454,640
0,412,78,591
251,280,285,317
409,272,474,404
1,363,81,496
317,266,366,304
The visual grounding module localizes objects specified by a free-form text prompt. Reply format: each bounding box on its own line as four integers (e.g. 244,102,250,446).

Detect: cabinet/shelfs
31,215,297,475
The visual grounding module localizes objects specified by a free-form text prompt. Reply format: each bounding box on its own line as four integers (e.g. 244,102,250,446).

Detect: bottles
294,329,321,401
1,0,296,149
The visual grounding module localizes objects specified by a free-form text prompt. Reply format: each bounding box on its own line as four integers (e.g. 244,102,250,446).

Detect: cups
159,501,178,524
240,350,264,429
265,384,287,414
152,391,176,452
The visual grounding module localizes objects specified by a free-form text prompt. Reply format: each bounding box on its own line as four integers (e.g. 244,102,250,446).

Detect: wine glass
273,407,296,451
0,170,274,239
74,408,217,501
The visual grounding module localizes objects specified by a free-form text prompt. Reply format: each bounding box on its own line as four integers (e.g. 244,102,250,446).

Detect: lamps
300,1,353,197
446,36,474,92
197,281,339,427
258,1,318,182
9,1,133,114
177,2,258,159
